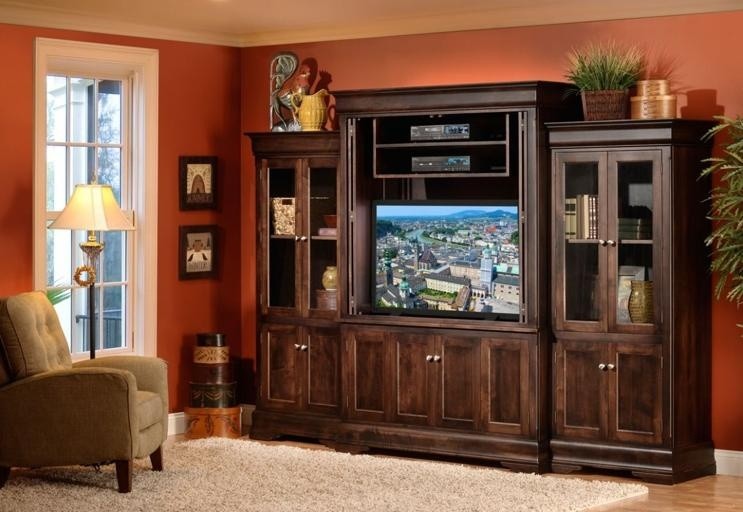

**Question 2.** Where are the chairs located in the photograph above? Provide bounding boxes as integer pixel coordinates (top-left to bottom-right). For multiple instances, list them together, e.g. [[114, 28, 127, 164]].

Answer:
[[0, 289, 172, 494]]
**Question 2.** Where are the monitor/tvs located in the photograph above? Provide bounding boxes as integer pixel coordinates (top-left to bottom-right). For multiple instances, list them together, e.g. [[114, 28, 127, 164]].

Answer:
[[370, 199, 521, 322]]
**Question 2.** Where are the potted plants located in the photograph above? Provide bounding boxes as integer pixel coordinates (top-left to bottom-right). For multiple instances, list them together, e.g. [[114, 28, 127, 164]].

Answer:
[[563, 34, 648, 121]]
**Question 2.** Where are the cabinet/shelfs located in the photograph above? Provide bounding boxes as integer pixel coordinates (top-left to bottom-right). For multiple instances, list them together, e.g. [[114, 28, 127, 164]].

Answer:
[[256, 321, 344, 419], [346, 324, 387, 425], [248, 133, 338, 319], [389, 326, 481, 432], [545, 120, 712, 334], [482, 330, 548, 439], [553, 336, 712, 452], [338, 80, 580, 332]]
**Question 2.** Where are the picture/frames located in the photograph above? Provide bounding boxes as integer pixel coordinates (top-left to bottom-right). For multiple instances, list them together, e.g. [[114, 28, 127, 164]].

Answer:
[[176, 153, 223, 283]]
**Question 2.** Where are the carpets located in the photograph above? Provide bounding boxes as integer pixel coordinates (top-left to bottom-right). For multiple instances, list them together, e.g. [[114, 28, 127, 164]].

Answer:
[[0, 433, 652, 511]]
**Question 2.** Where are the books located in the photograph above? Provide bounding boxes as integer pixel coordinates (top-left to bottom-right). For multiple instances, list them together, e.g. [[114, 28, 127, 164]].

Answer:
[[565, 193, 651, 240]]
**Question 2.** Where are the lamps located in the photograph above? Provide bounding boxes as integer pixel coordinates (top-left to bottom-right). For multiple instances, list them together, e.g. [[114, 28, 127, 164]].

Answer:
[[46, 182, 138, 361]]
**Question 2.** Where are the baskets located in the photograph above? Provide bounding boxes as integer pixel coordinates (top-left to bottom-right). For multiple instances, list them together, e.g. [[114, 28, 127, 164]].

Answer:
[[581, 90, 629, 122]]
